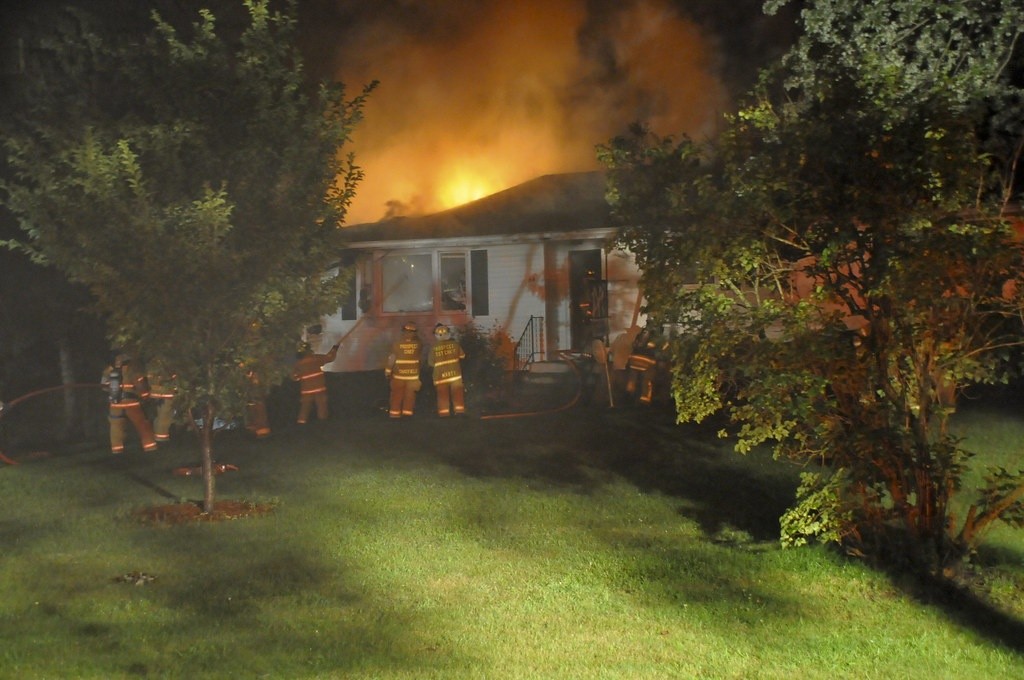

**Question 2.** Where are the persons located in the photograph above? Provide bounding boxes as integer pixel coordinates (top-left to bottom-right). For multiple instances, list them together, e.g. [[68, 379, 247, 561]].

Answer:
[[291, 342, 340, 422], [385, 321, 423, 419], [102, 349, 158, 454], [626, 328, 670, 405], [145, 350, 179, 441], [231, 348, 272, 435], [428, 325, 466, 419]]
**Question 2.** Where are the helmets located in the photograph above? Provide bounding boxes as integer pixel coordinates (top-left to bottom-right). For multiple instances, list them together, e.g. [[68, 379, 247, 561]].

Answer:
[[297, 342, 311, 352], [433, 322, 449, 336], [402, 321, 417, 332]]
